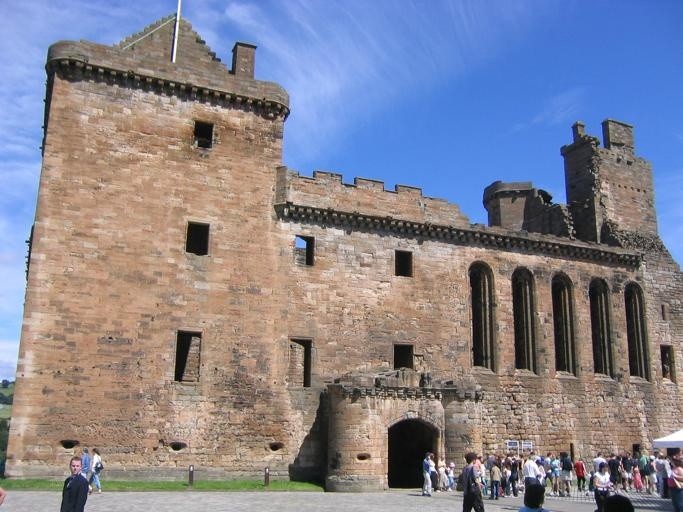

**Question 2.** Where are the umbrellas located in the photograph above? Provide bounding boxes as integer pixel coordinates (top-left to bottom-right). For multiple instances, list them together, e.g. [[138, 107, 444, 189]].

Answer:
[[652, 428, 682, 448]]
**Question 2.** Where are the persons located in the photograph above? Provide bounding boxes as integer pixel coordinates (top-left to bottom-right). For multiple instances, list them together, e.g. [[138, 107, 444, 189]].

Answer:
[[421, 447, 682, 512], [59, 456, 87, 512], [80, 447, 92, 495], [89, 447, 103, 494]]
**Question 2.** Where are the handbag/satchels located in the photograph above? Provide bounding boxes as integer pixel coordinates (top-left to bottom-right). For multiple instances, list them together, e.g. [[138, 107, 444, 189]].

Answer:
[[95, 462, 104, 473]]
